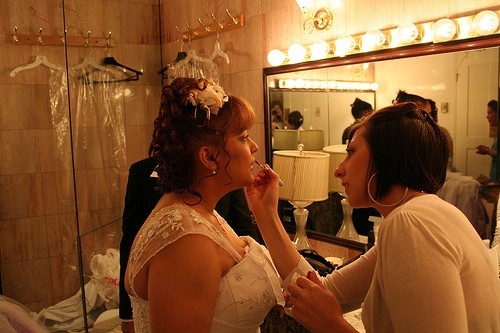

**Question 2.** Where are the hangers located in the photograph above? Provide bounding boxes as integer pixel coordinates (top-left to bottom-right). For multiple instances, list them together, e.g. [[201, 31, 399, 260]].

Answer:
[[9, 41, 65, 78], [167, 37, 215, 71], [77, 43, 143, 86], [71, 44, 122, 77], [157, 39, 202, 80], [209, 27, 230, 64]]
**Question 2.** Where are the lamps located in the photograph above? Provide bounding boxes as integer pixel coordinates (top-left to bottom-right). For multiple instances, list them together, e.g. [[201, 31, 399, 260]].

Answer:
[[299, 126, 324, 151], [322, 139, 360, 242], [272, 144, 330, 251], [273, 126, 299, 150]]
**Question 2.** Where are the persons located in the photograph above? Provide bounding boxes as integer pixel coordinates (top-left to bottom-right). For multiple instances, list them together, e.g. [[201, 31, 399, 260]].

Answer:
[[392, 91, 457, 172], [342, 98, 374, 144], [245, 102, 500, 333], [476, 100, 500, 180], [118, 77, 284, 333], [271, 100, 304, 131]]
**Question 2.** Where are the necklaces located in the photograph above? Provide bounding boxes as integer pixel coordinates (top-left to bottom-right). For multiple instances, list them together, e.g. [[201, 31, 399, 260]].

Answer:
[[399, 190, 425, 206], [182, 188, 227, 235]]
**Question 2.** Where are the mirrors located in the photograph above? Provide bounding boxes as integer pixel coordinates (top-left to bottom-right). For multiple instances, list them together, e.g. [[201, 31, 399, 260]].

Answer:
[[261, 45, 500, 265]]
